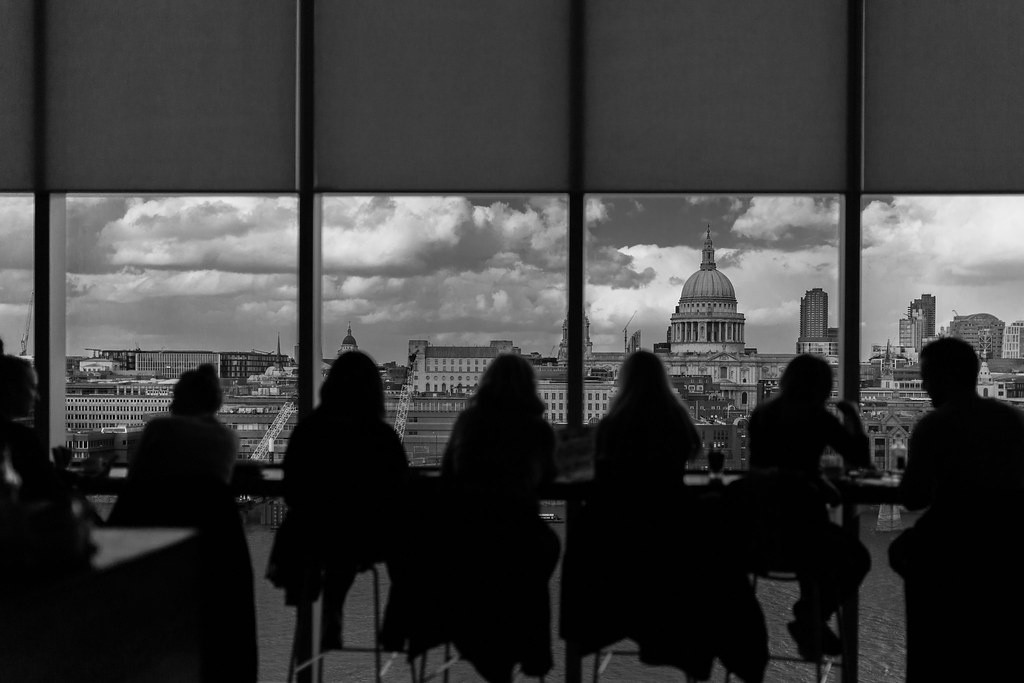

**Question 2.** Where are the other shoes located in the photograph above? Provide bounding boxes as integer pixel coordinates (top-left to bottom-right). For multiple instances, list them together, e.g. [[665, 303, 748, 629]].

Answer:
[[786, 616, 841, 664]]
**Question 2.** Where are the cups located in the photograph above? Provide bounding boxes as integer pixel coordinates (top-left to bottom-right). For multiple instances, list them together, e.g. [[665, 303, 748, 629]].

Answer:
[[83, 458, 102, 472], [707, 448, 726, 479]]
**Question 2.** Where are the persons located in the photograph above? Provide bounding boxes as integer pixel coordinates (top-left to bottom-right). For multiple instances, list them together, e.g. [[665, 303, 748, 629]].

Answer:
[[127, 362, 236, 483], [747, 354, 870, 663], [888, 339, 1024, 579], [594, 350, 702, 497], [0, 355, 74, 502], [442, 354, 561, 582], [283, 350, 409, 501]]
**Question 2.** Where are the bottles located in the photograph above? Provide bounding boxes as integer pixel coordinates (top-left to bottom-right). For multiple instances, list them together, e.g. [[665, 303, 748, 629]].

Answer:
[[888, 432, 906, 474]]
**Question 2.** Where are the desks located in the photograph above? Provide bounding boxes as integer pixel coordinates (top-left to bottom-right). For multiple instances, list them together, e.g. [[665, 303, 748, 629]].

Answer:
[[55, 460, 904, 681]]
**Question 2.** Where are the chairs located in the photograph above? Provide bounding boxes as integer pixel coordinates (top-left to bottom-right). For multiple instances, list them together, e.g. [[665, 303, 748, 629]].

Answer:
[[280, 547, 835, 682]]
